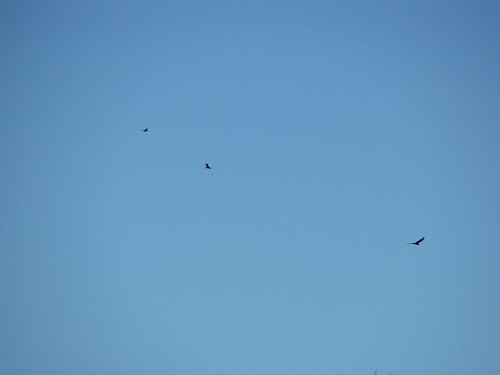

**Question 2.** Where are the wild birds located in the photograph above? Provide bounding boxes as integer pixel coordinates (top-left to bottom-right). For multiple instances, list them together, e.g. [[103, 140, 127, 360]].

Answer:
[[140, 127, 148, 133], [406, 235, 425, 245], [204, 163, 211, 170]]
